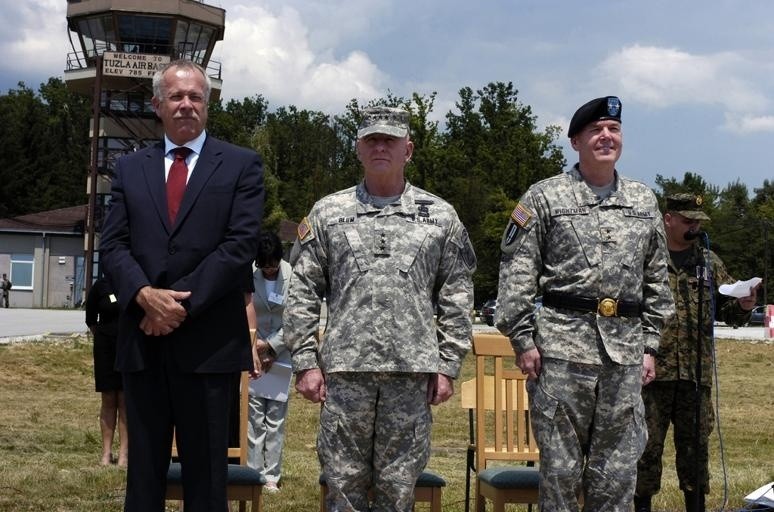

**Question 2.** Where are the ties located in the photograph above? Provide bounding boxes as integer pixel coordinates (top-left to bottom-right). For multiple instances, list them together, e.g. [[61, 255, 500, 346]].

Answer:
[[166, 149, 190, 227]]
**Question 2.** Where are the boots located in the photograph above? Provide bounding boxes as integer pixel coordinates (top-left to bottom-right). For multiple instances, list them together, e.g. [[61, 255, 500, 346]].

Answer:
[[633, 492, 652, 512], [684, 491, 706, 512]]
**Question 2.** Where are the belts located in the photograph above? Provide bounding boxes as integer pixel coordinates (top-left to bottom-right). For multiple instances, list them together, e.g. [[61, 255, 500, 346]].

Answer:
[[540, 289, 643, 317]]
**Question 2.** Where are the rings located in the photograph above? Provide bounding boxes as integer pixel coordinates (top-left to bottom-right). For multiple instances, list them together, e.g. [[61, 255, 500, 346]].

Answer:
[[521, 371, 529, 375]]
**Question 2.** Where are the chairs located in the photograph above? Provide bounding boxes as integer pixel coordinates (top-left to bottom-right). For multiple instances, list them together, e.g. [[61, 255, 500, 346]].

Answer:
[[460, 374, 538, 511], [470, 333, 587, 511], [162, 326, 269, 512], [318, 332, 446, 511]]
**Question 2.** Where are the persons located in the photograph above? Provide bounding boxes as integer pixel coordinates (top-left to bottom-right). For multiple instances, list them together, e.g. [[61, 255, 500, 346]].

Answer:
[[280, 108, 478, 512], [0, 274, 12, 308], [494, 94, 676, 512], [632, 192, 762, 512], [84, 271, 129, 469], [97, 62, 264, 512], [246, 229, 293, 495]]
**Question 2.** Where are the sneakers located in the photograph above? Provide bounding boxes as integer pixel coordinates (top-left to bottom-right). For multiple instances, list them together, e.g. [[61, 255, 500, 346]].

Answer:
[[262, 480, 279, 493]]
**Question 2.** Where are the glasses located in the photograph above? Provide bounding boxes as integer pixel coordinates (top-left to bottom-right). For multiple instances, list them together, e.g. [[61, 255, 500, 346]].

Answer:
[[254, 263, 279, 271]]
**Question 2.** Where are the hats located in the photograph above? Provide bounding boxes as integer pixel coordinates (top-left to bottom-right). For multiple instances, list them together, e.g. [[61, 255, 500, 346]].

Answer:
[[567, 94, 624, 139], [666, 191, 712, 224], [356, 105, 411, 141]]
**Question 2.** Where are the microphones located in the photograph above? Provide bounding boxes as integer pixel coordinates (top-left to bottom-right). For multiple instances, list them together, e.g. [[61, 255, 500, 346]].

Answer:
[[683, 229, 708, 241]]
